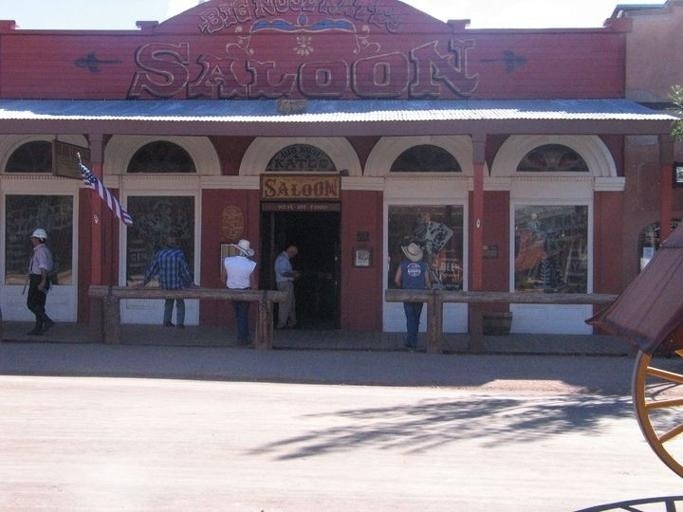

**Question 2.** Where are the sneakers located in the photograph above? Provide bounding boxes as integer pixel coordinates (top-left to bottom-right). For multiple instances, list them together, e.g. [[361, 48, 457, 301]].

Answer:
[[239, 335, 253, 344], [405, 344, 417, 351], [163, 320, 184, 328], [279, 324, 301, 329]]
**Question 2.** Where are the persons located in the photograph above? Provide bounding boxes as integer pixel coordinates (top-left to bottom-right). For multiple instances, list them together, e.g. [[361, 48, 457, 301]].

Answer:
[[413, 211, 455, 285], [394, 243, 432, 348], [223, 238, 258, 343], [128, 232, 200, 329], [25, 227, 56, 336], [273, 244, 301, 330]]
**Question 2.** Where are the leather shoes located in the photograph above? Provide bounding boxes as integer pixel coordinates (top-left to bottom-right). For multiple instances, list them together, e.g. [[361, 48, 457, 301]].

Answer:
[[32, 315, 53, 333]]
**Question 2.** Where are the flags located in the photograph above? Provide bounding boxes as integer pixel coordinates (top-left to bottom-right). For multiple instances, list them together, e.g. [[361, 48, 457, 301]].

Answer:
[[78, 162, 133, 229]]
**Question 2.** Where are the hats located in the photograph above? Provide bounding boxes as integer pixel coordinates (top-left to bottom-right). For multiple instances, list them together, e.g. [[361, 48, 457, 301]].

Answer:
[[400, 243, 423, 262], [233, 239, 255, 256], [32, 228, 48, 239]]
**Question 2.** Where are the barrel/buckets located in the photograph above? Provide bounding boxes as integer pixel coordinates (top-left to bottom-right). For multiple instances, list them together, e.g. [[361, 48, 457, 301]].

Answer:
[[484, 311, 513, 334]]
